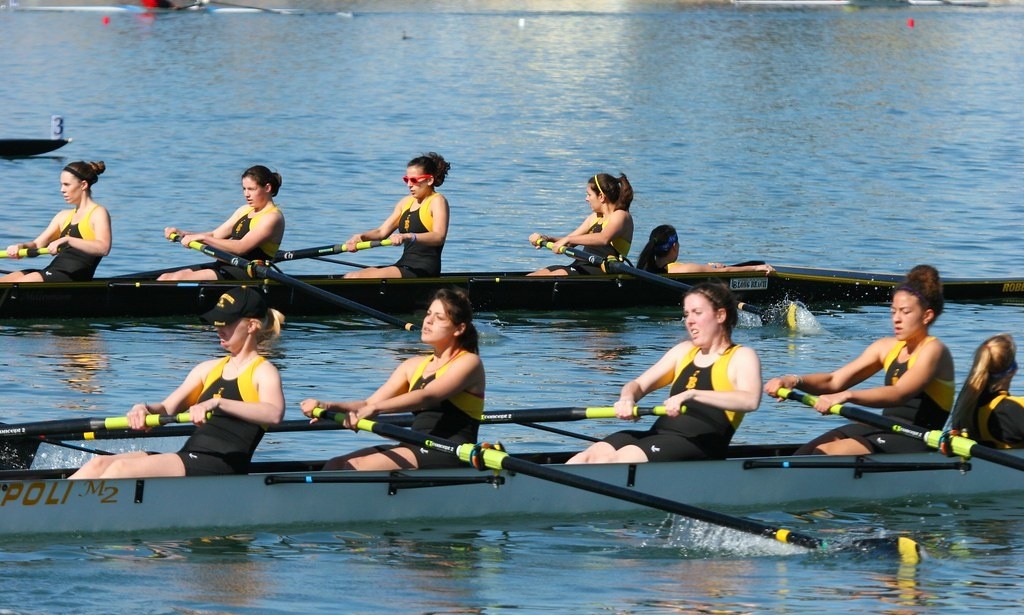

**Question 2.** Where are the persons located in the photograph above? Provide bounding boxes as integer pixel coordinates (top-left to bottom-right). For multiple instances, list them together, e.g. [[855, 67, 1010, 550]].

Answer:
[[763, 263, 956, 456], [155, 165, 285, 281], [636, 224, 776, 273], [945, 333, 1024, 450], [524, 172, 633, 276], [0, 160, 112, 284], [301, 288, 487, 472], [65, 288, 287, 480], [564, 283, 763, 465], [340, 152, 451, 278]]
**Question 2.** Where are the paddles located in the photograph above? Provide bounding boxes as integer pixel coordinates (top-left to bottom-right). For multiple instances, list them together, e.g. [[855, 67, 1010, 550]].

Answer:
[[482, 403, 687, 423], [1, 245, 62, 258], [312, 255, 386, 268], [1, 410, 214, 437], [537, 239, 805, 330], [776, 386, 1023, 472], [298, 399, 918, 576], [713, 260, 765, 266], [277, 240, 392, 262], [168, 234, 421, 333]]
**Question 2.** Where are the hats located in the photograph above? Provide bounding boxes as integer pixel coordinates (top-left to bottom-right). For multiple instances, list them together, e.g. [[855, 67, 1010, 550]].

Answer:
[[199, 286, 266, 326]]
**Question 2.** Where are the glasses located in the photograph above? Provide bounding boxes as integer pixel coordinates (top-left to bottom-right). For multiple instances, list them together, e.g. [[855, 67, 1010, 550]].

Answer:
[[401, 175, 430, 183]]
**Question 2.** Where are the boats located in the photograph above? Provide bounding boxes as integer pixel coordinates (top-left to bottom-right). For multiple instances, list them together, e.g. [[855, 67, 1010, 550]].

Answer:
[[0, 259, 1024, 319], [0, 137, 72, 159], [14, 5, 355, 20], [2, 432, 1023, 539]]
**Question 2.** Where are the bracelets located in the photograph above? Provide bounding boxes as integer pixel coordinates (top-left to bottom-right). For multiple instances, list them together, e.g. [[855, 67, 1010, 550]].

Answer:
[[785, 373, 803, 389], [408, 232, 416, 243], [708, 262, 716, 269], [543, 234, 549, 242]]
